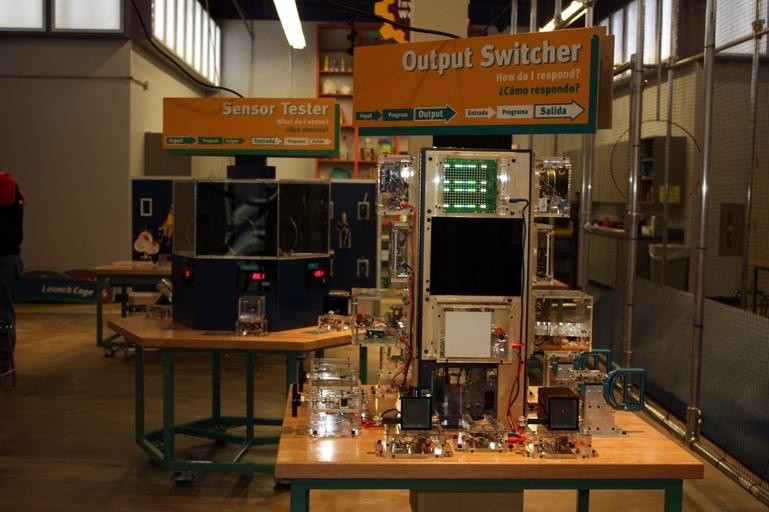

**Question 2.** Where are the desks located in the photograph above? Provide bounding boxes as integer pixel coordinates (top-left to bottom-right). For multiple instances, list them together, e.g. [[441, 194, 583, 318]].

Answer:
[[533, 287, 600, 376], [355, 312, 413, 381], [274, 377, 703, 508], [107, 308, 352, 489], [96, 264, 172, 356]]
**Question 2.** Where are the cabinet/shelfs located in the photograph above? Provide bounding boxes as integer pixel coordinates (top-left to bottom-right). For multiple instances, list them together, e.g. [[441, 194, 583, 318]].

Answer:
[[639, 136, 687, 207], [587, 234, 656, 288], [314, 23, 400, 181]]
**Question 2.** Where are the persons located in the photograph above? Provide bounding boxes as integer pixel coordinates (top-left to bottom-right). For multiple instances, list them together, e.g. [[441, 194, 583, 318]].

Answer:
[[0, 171, 23, 377]]
